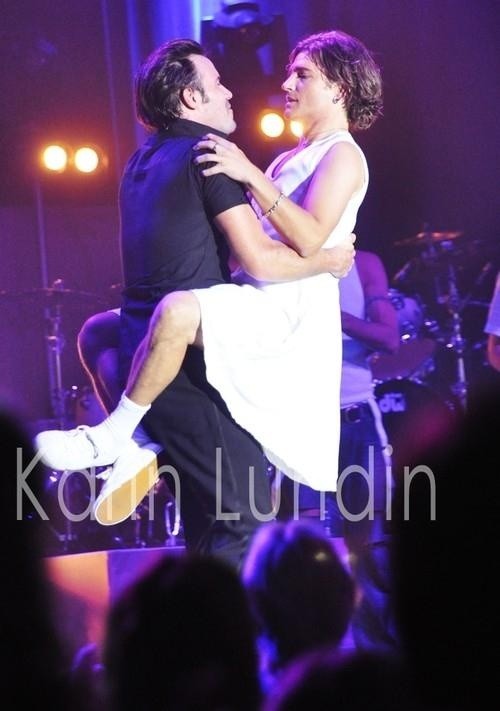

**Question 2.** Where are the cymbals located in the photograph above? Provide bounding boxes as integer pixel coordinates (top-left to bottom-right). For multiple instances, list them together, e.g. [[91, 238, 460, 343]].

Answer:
[[0, 288, 108, 310], [396, 231, 462, 247]]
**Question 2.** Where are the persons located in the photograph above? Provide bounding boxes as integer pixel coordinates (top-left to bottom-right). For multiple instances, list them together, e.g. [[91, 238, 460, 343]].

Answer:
[[32, 26, 388, 527], [475, 272, 500, 376], [0, 365, 499, 711], [116, 33, 357, 584], [266, 247, 403, 650]]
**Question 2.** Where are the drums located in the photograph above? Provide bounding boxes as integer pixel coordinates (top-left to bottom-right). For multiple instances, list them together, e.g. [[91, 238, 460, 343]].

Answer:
[[367, 287, 440, 377], [372, 371, 461, 476], [75, 385, 102, 425]]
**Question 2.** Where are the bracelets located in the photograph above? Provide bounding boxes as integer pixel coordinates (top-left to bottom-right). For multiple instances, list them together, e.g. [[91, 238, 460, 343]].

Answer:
[[260, 194, 289, 218]]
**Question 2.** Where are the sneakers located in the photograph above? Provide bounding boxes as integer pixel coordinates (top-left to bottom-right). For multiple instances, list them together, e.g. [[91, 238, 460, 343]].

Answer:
[[32, 423, 120, 471], [92, 448, 158, 525]]
